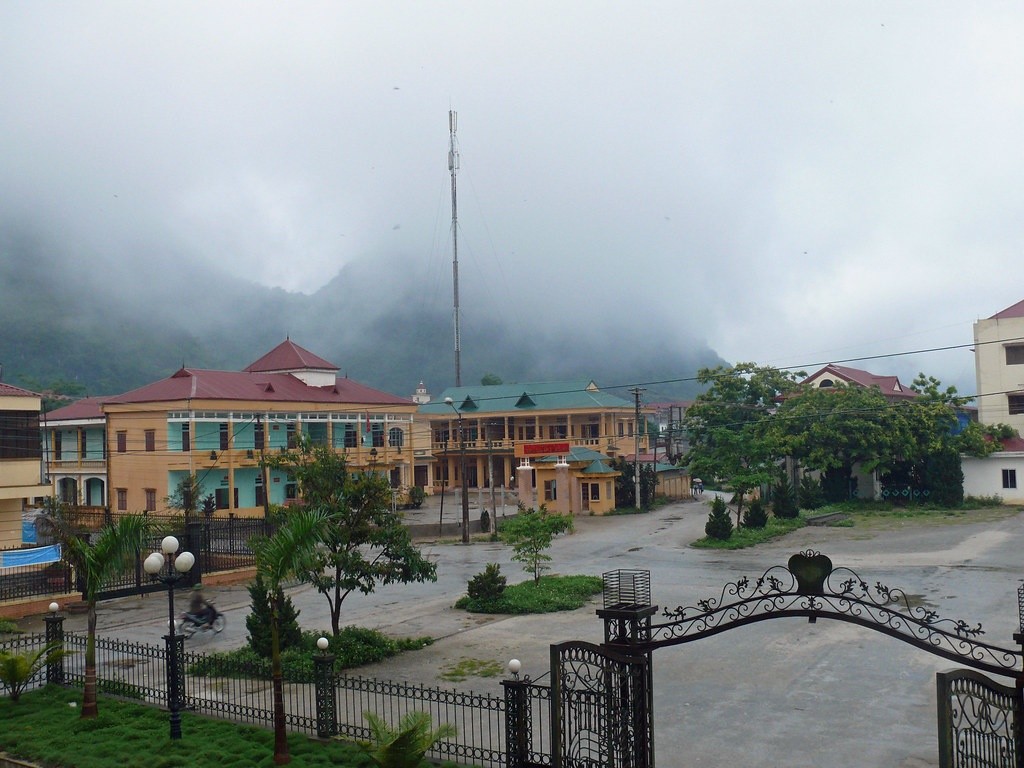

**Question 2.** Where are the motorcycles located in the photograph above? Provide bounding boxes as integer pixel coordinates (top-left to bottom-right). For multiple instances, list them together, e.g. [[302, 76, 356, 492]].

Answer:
[[176, 596, 227, 641]]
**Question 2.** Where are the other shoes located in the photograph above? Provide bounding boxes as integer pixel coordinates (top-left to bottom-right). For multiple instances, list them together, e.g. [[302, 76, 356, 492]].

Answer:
[[206, 626, 212, 629]]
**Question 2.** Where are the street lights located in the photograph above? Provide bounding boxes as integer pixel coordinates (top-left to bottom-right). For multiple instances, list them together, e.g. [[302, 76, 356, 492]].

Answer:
[[445, 397, 472, 543], [142, 535, 195, 737]]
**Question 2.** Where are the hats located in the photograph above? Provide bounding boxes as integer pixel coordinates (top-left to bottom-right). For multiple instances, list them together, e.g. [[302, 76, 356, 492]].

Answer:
[[192, 583, 203, 590]]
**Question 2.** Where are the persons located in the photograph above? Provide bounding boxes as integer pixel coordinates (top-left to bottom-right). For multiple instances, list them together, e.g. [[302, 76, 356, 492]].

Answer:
[[690, 481, 704, 496], [190, 583, 216, 629]]
